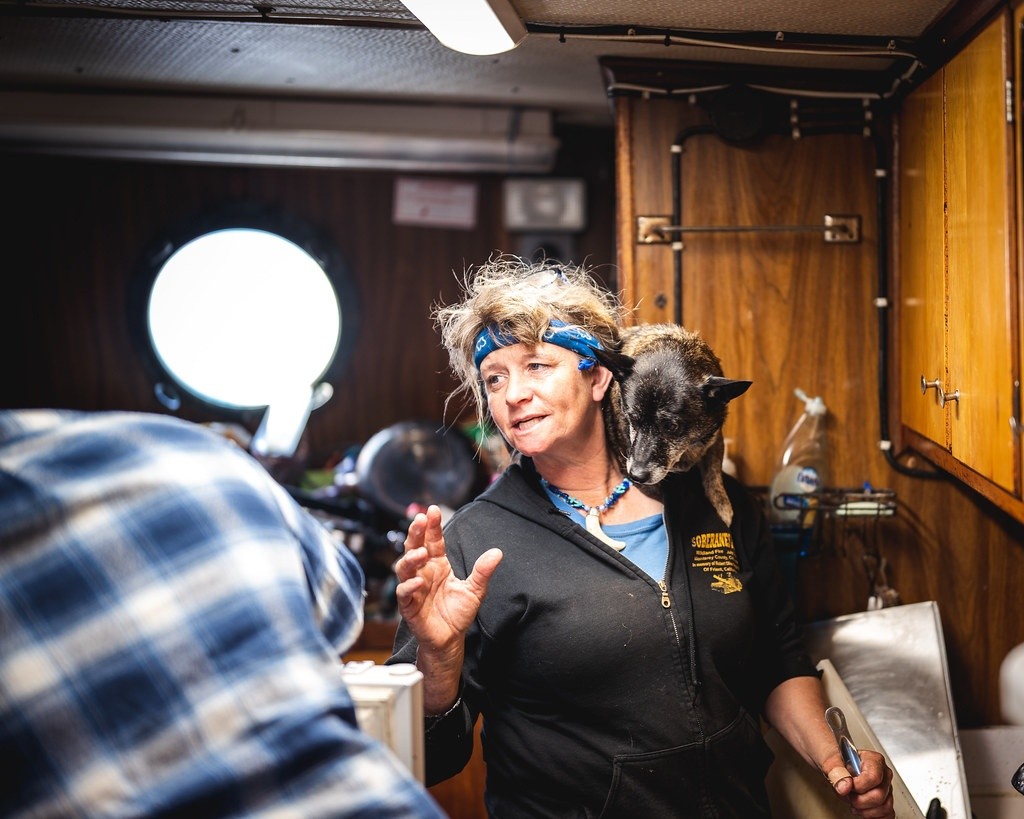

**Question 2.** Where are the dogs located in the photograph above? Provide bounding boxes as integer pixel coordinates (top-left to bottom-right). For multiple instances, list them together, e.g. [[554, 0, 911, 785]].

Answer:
[[603, 320, 754, 530]]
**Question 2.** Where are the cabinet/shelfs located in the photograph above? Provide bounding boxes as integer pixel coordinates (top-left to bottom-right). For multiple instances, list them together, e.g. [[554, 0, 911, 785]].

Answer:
[[884, 15, 1021, 515]]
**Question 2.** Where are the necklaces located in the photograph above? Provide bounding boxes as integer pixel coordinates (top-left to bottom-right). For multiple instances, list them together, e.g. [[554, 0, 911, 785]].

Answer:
[[536, 473, 633, 551]]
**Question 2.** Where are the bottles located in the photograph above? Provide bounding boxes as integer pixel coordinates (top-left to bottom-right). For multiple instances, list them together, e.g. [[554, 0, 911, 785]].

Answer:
[[721, 437, 736, 477], [763, 388, 829, 556]]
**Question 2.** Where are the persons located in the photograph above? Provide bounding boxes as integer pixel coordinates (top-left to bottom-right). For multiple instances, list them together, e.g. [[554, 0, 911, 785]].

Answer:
[[2, 403, 448, 819], [387, 253, 898, 819]]
